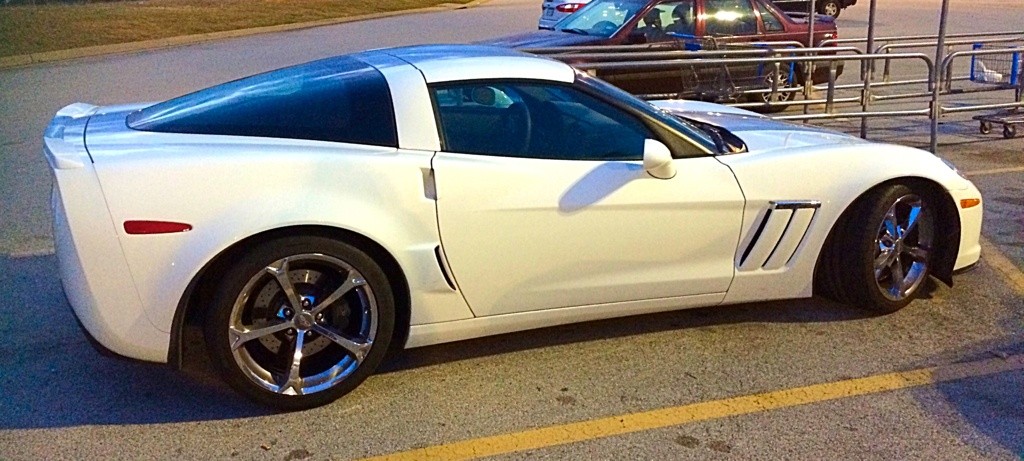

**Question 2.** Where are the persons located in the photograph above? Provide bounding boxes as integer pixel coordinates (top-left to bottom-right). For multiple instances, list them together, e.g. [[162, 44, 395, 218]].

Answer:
[[435, 94, 501, 155]]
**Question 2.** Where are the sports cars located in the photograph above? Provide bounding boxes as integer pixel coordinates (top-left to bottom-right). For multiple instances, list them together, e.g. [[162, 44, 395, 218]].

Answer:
[[40, 44, 985, 410]]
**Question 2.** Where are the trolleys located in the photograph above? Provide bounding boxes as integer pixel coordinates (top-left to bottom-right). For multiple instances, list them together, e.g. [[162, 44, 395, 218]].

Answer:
[[967, 42, 1024, 139], [664, 31, 796, 104]]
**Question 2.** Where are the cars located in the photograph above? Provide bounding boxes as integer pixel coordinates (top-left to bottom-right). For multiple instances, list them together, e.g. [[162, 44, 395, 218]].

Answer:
[[537, 0, 616, 31], [769, 0, 859, 20], [447, 1, 846, 103]]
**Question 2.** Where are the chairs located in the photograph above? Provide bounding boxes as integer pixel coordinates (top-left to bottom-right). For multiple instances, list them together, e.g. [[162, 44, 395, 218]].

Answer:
[[663, 4, 690, 37]]
